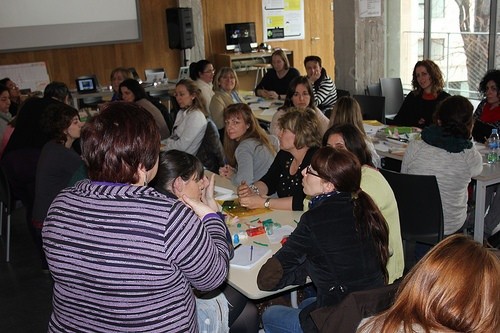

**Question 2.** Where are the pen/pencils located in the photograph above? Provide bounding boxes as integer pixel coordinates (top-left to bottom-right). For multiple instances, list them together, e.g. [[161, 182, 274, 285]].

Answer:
[[484, 137, 489, 142], [404, 131, 409, 138], [250, 246, 253, 260], [294, 220, 298, 224], [386, 136, 398, 140], [383, 142, 393, 148], [234, 244, 242, 250]]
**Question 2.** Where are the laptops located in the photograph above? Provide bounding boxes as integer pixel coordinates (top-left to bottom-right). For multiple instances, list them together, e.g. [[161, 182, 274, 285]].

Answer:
[[76, 78, 97, 93]]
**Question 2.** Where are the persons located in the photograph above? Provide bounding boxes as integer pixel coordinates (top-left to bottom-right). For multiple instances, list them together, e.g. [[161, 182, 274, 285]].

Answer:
[[470, 69, 500, 144], [401, 95, 483, 238], [0, 50, 405, 333], [356, 234, 500, 333], [389, 59, 450, 129]]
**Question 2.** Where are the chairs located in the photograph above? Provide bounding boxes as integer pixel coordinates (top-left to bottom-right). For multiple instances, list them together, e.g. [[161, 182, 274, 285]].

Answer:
[[378, 167, 444, 245], [0, 166, 24, 262], [148, 95, 181, 133], [320, 284, 396, 333], [197, 120, 226, 174], [352, 94, 386, 126], [365, 77, 404, 124]]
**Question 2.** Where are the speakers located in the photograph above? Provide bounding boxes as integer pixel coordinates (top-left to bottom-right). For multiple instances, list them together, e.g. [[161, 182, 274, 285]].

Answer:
[[166, 8, 194, 50]]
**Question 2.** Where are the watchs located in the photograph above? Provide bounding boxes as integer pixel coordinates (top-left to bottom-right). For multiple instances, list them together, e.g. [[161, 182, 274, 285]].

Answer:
[[264, 198, 272, 210]]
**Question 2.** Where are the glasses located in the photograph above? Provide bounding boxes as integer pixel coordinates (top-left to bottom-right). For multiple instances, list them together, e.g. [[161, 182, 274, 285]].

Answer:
[[306, 166, 324, 180], [173, 92, 190, 97], [204, 70, 215, 75], [10, 83, 20, 89]]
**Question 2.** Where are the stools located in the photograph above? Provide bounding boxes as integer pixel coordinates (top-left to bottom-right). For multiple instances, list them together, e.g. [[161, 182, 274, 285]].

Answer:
[[252, 63, 273, 89]]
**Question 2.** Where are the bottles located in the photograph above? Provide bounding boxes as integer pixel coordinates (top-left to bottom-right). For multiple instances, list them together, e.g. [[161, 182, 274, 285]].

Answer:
[[487, 129, 499, 164]]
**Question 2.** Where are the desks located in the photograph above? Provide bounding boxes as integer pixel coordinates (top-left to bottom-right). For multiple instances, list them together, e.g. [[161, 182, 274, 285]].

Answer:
[[203, 169, 311, 308], [250, 99, 500, 247], [69, 82, 176, 110], [216, 50, 294, 72]]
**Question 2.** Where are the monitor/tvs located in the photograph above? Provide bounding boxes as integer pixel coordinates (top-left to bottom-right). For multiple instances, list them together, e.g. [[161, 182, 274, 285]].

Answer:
[[147, 72, 163, 86], [224, 22, 258, 50]]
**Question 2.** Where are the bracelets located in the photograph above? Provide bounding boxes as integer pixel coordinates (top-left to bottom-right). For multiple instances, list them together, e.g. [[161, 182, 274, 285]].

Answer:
[[278, 94, 281, 100], [248, 185, 260, 196]]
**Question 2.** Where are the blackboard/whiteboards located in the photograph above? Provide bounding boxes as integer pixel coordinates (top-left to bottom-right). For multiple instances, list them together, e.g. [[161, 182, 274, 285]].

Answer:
[[0, 62, 51, 97]]
